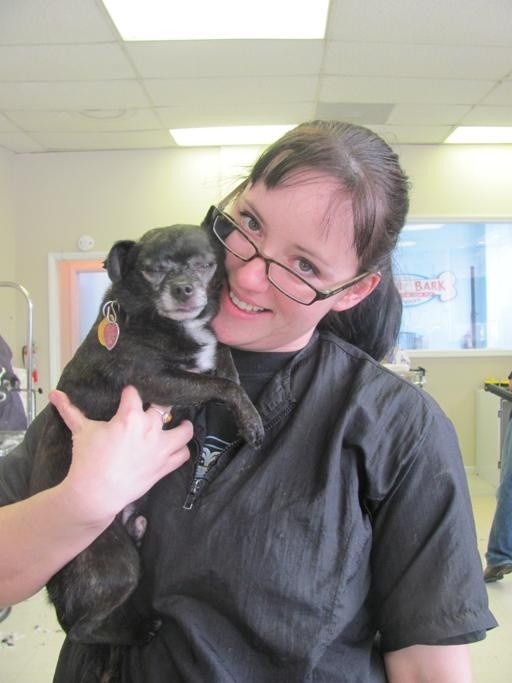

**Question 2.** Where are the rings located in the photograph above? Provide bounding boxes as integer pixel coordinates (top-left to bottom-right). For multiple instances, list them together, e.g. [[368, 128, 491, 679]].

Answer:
[[150, 405, 173, 424]]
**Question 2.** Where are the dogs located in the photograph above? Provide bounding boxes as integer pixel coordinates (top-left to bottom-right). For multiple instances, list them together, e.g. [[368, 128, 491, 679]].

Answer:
[[27, 205, 266, 683]]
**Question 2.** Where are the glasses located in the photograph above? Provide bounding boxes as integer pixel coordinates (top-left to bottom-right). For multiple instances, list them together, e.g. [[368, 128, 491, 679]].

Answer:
[[212, 177, 371, 306]]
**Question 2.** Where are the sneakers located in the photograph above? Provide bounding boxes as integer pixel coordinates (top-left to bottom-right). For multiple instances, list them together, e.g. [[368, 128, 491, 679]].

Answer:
[[484, 563, 512, 583]]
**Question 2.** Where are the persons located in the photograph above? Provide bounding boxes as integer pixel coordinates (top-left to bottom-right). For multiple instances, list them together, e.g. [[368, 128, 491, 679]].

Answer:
[[484, 369, 512, 582], [0, 119, 499, 683]]
